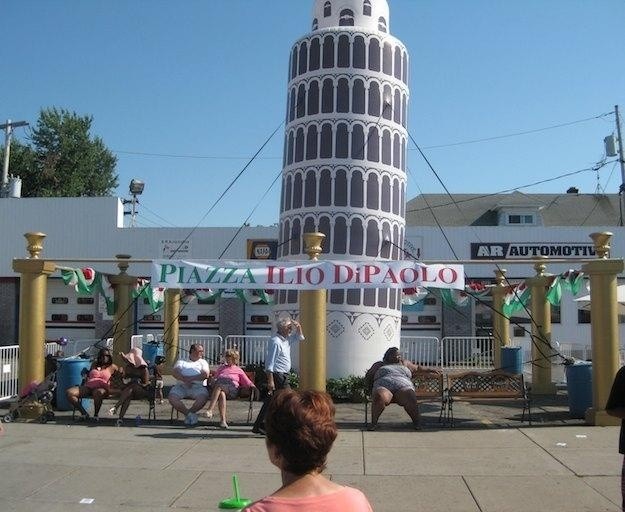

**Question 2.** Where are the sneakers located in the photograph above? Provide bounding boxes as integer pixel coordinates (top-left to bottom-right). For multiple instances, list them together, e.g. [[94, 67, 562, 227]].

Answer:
[[220, 422, 228, 428], [185, 411, 212, 424], [79, 414, 99, 423]]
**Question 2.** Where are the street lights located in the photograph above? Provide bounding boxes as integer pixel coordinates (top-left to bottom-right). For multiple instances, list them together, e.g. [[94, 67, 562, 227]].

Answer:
[[130, 178, 145, 226]]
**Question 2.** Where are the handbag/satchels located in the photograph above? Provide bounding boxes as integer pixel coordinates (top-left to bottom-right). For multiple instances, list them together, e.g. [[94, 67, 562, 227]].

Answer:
[[207, 377, 215, 397]]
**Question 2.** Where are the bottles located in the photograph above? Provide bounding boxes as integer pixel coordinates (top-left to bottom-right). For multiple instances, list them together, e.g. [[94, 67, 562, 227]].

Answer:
[[134, 414, 142, 427]]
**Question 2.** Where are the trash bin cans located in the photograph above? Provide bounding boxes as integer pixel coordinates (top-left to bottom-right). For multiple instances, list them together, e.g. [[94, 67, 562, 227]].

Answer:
[[142, 342, 165, 376], [500, 346, 523, 374], [565, 364, 593, 419], [45, 354, 91, 411]]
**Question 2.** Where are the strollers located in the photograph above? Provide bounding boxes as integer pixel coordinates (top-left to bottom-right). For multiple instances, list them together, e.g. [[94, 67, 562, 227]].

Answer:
[[1, 370, 57, 423]]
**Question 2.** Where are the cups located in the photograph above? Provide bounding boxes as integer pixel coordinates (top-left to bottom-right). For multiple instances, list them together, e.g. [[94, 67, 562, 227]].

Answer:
[[217, 498, 252, 512]]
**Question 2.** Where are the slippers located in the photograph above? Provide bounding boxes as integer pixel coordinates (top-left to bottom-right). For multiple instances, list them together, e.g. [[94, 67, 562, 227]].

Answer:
[[107, 407, 125, 427]]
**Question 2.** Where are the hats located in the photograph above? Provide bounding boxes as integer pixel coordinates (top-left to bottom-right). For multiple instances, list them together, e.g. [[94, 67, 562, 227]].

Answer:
[[119, 347, 148, 368]]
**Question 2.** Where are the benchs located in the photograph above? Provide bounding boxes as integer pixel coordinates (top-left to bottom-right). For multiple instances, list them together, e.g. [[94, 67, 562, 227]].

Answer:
[[71, 363, 255, 429], [363, 370, 532, 428]]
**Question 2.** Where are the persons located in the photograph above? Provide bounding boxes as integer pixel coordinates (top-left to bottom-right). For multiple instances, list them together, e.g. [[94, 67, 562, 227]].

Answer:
[[147, 356, 167, 404], [65, 348, 124, 423], [364, 346, 443, 432], [167, 343, 210, 427], [106, 347, 150, 427], [604, 365, 624, 512], [251, 311, 305, 435], [241, 388, 372, 512], [200, 349, 260, 431]]
